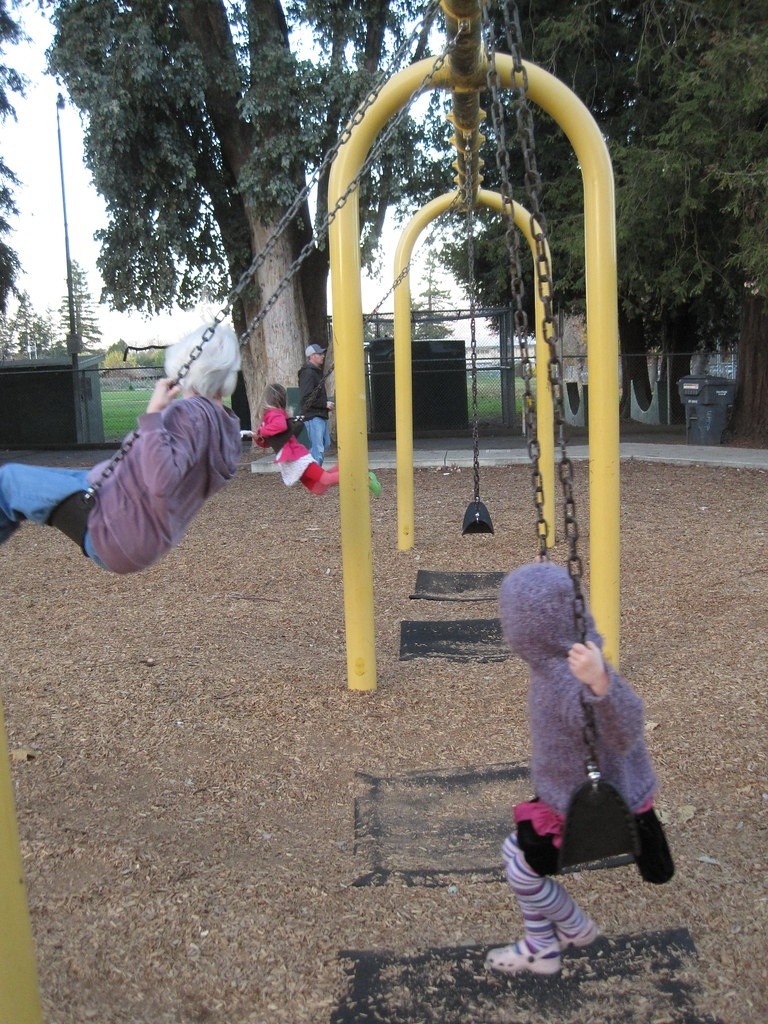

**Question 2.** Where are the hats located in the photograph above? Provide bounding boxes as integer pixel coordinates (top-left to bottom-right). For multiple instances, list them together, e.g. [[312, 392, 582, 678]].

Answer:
[[305, 343, 327, 358]]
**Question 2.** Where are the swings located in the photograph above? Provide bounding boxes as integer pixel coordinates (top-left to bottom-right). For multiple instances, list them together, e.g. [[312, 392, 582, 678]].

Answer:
[[459, 124, 496, 540], [45, 1, 472, 560], [476, 0, 642, 876], [266, 190, 473, 454]]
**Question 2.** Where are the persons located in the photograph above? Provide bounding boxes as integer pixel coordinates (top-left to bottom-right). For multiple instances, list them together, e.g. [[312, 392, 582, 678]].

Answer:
[[255, 383, 383, 499], [1, 324, 245, 573], [296, 343, 334, 468], [484, 563, 655, 974]]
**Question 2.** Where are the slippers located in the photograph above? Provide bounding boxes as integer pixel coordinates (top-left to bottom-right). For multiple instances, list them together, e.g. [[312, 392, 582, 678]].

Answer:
[[368, 472, 382, 497], [552, 919, 598, 948], [486, 937, 562, 975]]
